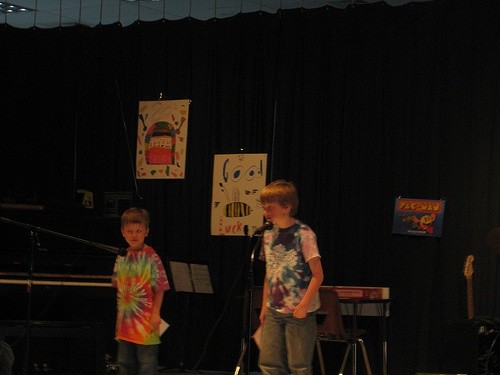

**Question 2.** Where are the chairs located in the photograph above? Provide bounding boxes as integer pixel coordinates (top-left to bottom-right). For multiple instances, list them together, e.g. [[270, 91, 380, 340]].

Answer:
[[316, 288, 373, 375]]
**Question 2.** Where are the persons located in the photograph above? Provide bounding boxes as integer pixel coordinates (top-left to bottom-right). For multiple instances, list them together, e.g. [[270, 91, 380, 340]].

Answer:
[[112, 208, 170, 375], [259, 180, 324, 375]]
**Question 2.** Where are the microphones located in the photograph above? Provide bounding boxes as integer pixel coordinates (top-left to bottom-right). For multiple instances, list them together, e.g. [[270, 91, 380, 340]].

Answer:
[[98, 244, 127, 256], [253, 222, 273, 235]]
